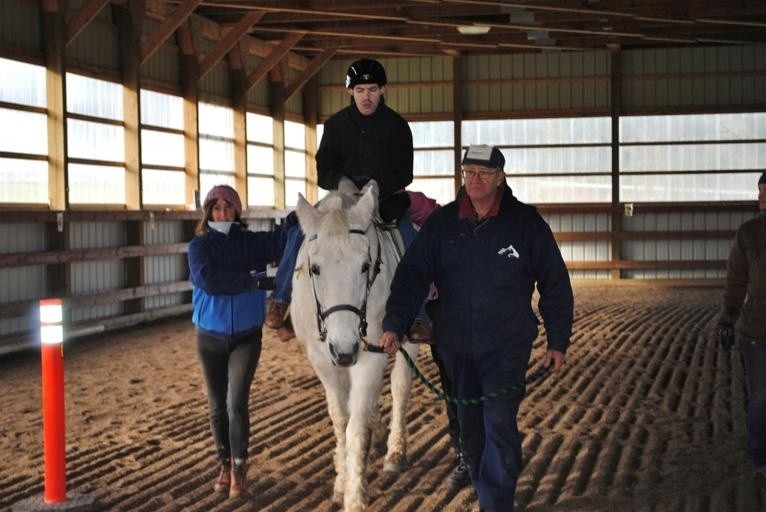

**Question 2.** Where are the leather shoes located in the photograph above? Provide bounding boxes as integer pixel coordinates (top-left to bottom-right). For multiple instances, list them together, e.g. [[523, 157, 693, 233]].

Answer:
[[447, 462, 468, 490]]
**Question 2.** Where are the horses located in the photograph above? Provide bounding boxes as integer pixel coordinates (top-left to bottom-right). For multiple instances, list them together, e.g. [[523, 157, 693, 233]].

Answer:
[[289, 186, 421, 512]]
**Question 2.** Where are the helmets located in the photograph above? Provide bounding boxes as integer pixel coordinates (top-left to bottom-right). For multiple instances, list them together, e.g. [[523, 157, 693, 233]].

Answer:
[[346, 59, 386, 89]]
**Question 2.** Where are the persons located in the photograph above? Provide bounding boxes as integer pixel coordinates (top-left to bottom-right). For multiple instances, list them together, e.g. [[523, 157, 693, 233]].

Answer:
[[376, 183, 471, 488], [262, 57, 433, 345], [184, 182, 299, 502], [714, 166, 766, 493], [375, 142, 574, 511]]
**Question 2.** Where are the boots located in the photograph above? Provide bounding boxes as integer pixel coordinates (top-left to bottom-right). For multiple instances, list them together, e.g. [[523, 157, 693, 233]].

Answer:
[[214, 459, 230, 494], [229, 457, 253, 503]]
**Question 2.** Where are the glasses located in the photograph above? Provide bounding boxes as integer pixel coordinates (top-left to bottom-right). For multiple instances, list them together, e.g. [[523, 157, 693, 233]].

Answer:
[[460, 168, 501, 180]]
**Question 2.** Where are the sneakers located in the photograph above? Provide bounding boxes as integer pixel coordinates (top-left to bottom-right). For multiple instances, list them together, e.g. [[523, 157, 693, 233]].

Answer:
[[409, 320, 431, 345], [265, 300, 290, 330]]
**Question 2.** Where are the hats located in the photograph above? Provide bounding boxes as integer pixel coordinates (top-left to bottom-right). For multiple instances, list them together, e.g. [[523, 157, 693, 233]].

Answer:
[[458, 144, 504, 173], [203, 185, 242, 217]]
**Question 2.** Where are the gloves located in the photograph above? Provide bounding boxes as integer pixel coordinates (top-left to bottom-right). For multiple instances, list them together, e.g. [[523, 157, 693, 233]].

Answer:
[[716, 319, 736, 350]]
